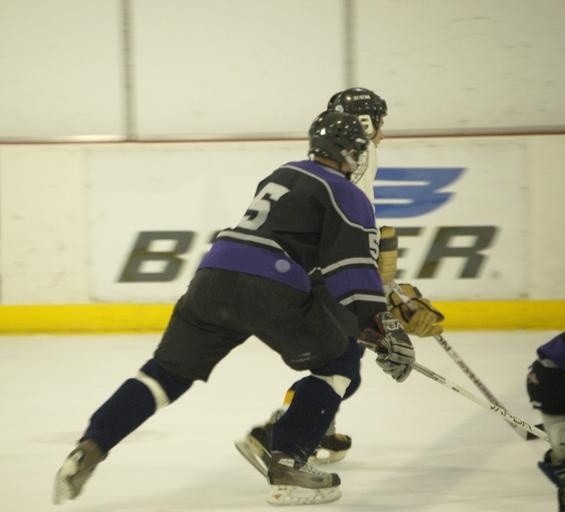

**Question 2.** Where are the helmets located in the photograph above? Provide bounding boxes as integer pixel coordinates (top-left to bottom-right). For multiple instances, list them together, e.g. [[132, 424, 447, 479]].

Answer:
[[308, 110, 370, 163], [333, 88, 388, 132]]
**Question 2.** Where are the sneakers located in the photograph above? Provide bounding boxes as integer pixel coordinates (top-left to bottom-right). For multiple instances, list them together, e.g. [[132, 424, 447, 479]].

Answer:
[[269, 449, 342, 490], [64, 440, 103, 500], [252, 408, 286, 469], [313, 432, 353, 452]]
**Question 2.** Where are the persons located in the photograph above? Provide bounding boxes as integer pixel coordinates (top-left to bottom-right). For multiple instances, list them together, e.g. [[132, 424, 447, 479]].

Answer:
[[526, 333, 565, 512], [235, 87, 445, 479], [49, 109, 415, 507]]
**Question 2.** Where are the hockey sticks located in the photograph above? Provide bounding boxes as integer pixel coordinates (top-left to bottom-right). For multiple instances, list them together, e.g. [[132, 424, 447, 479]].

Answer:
[[390, 279, 545, 440]]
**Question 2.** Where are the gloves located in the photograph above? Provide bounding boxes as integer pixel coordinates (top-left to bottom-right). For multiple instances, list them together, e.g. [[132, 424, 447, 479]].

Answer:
[[392, 282, 446, 337], [359, 312, 416, 383]]
